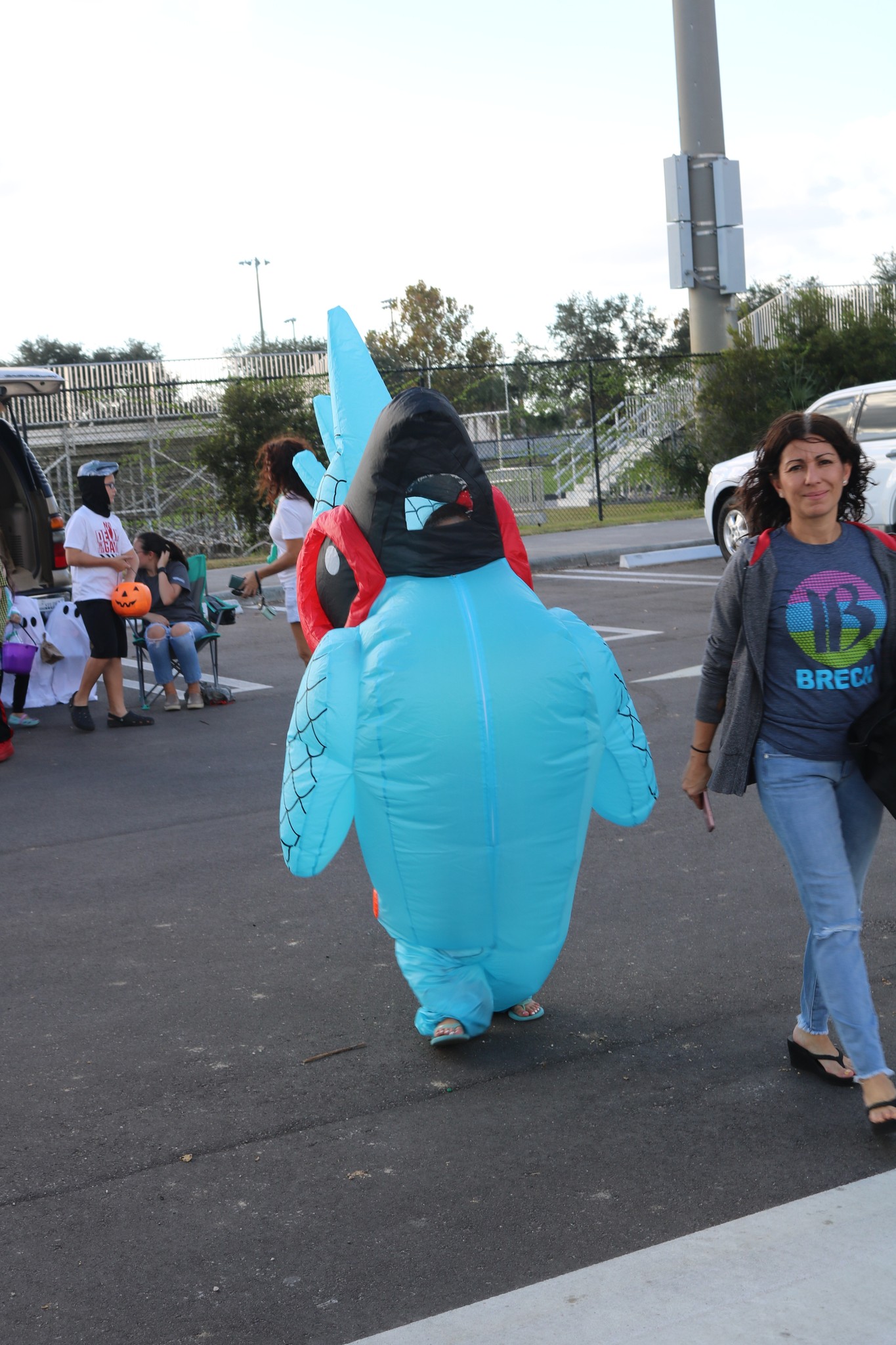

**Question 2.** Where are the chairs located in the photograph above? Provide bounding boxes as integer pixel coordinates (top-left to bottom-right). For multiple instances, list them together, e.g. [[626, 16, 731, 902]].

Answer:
[[135, 554, 220, 712]]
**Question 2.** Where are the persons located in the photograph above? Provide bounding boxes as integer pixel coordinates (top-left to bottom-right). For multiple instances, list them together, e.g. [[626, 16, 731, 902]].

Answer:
[[238, 438, 317, 667], [0, 556, 40, 761], [133, 532, 209, 710], [63, 460, 155, 731], [681, 413, 896, 1139], [279, 306, 658, 1043]]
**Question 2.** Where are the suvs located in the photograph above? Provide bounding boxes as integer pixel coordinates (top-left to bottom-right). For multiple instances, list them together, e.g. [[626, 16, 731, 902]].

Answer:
[[704, 379, 896, 563]]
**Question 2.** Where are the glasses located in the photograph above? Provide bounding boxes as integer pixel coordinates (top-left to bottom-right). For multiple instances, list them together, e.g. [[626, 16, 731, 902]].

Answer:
[[104, 481, 116, 489]]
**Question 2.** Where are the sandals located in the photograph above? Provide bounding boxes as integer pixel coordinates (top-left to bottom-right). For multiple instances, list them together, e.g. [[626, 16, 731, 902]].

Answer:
[[509, 997, 545, 1021], [431, 1017, 469, 1044], [69, 690, 95, 732], [107, 710, 155, 728]]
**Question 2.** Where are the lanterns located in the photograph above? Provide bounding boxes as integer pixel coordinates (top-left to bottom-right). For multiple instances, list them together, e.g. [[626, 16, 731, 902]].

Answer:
[[112, 582, 152, 617]]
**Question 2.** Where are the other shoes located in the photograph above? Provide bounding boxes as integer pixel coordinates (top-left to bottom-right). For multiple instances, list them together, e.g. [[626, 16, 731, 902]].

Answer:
[[7, 713, 40, 726]]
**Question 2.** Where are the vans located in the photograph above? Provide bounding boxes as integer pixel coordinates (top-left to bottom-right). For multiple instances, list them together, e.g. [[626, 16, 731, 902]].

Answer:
[[1, 366, 72, 614]]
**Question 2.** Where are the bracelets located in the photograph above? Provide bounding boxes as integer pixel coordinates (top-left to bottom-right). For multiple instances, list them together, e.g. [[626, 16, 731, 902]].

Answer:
[[156, 567, 167, 573], [690, 745, 710, 753]]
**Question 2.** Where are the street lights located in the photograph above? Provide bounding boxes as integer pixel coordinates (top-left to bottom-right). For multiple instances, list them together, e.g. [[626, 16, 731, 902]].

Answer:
[[285, 317, 297, 341], [238, 256, 271, 385], [381, 298, 397, 348]]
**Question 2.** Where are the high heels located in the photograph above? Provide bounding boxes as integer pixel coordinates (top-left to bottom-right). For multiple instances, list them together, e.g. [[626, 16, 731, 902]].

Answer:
[[785, 1035, 856, 1085], [863, 1089, 896, 1135]]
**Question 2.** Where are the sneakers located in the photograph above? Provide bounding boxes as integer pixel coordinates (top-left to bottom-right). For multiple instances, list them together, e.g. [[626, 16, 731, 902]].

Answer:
[[163, 694, 182, 710], [186, 691, 204, 708]]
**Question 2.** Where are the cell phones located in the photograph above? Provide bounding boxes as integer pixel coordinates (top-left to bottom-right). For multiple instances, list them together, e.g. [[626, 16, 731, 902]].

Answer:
[[701, 791, 715, 832]]
